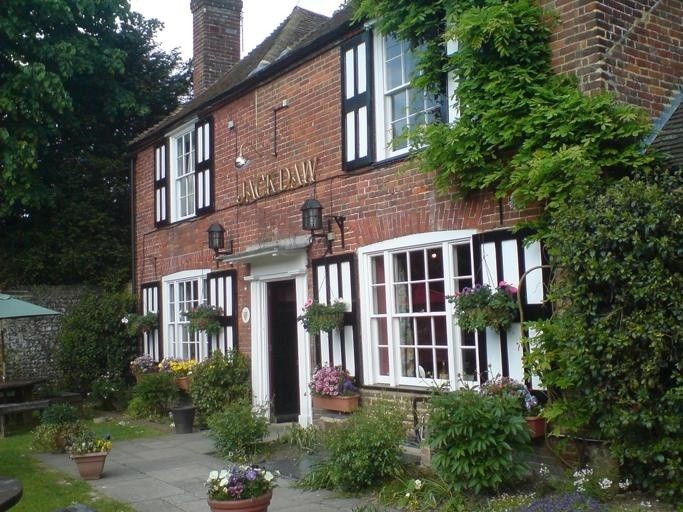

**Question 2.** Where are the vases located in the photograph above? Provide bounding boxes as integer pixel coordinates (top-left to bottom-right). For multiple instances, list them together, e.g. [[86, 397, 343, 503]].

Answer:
[[311, 394, 360, 416], [313, 313, 344, 329], [71, 452, 108, 480], [524, 417, 547, 437], [206, 490, 273, 512], [170, 406, 196, 434], [137, 376, 191, 390]]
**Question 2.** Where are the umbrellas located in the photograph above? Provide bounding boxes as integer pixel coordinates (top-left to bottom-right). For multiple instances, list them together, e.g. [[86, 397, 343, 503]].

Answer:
[[1, 295, 64, 320]]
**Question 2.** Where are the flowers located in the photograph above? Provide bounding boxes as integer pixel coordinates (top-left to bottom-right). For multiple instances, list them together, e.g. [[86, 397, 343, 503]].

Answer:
[[120, 312, 158, 337], [180, 304, 223, 336], [296, 298, 345, 337], [309, 363, 354, 396], [129, 353, 196, 376], [203, 465, 274, 501], [447, 281, 517, 334], [478, 378, 538, 411], [65, 431, 112, 456]]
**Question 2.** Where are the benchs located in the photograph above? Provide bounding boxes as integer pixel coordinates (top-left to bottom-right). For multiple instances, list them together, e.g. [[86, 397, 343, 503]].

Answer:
[[51, 393, 85, 416], [0, 399, 52, 437]]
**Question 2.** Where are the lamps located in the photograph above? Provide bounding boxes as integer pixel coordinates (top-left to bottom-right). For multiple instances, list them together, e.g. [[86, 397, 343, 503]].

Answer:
[[235, 99, 289, 169], [299, 198, 344, 248], [207, 224, 234, 257]]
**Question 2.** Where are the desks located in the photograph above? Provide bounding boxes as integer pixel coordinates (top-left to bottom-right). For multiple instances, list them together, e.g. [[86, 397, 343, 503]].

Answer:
[[0, 380, 46, 402]]
